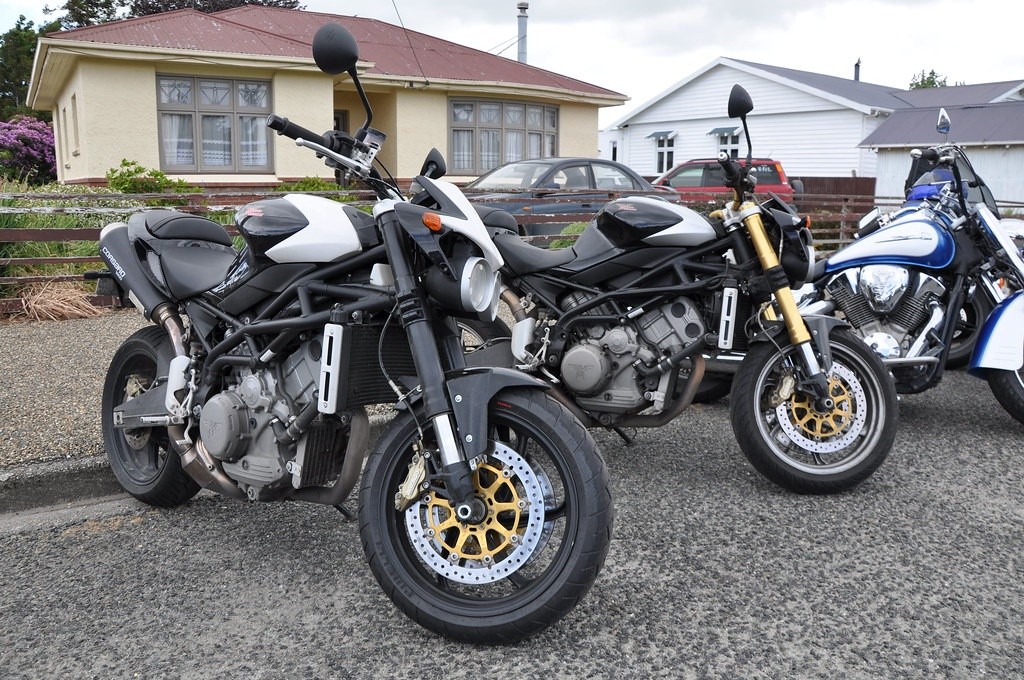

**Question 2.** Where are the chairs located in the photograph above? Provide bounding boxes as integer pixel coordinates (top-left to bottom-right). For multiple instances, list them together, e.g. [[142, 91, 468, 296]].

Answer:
[[538, 174, 633, 189]]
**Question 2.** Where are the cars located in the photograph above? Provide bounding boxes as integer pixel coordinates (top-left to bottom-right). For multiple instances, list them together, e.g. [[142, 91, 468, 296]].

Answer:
[[456, 156, 659, 224]]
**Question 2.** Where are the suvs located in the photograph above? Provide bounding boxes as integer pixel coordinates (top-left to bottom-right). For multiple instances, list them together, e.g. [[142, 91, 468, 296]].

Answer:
[[648, 155, 804, 219]]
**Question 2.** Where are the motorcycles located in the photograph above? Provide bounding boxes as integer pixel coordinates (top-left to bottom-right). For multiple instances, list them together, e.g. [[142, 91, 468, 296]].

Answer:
[[430, 82, 902, 494], [85, 22, 618, 646], [689, 107, 1023, 423]]
